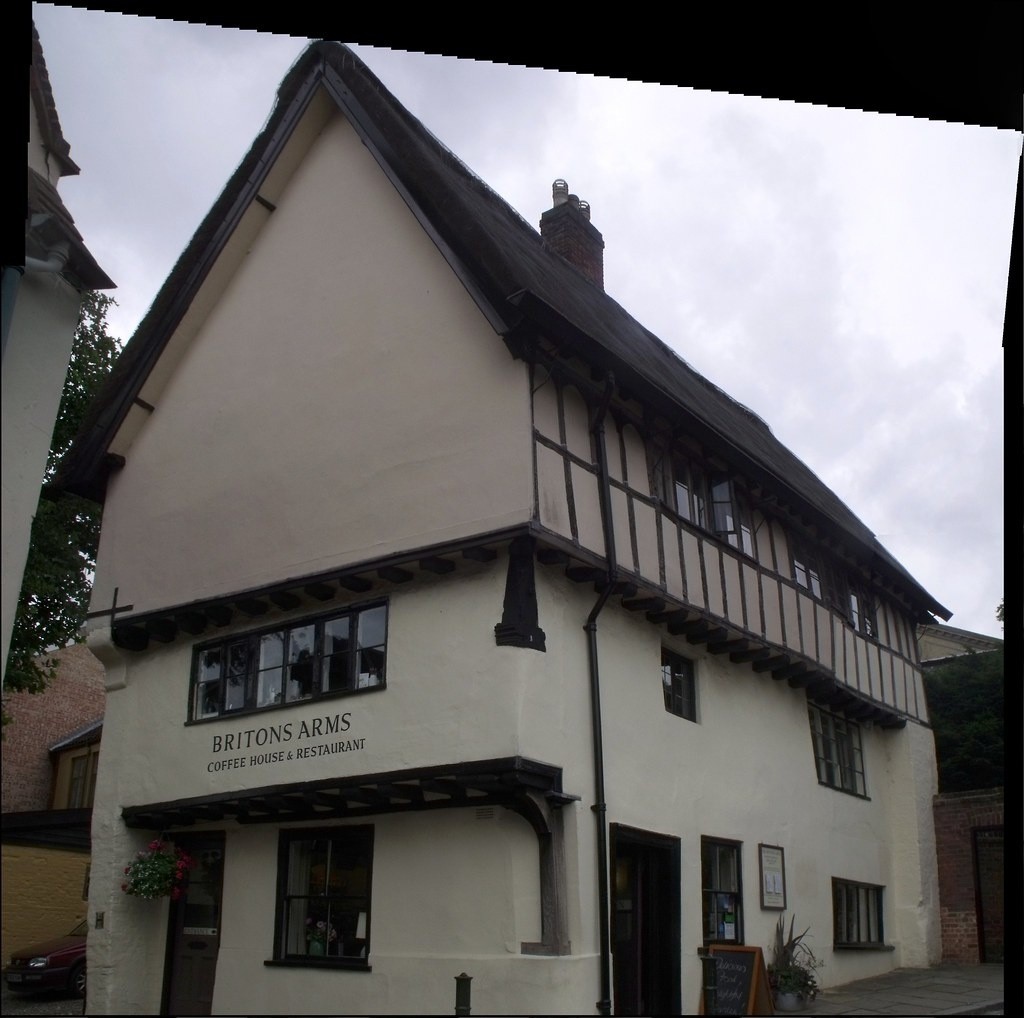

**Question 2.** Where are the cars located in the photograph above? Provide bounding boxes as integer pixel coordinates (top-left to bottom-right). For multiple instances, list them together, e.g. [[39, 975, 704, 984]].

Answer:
[[3, 921, 85, 998]]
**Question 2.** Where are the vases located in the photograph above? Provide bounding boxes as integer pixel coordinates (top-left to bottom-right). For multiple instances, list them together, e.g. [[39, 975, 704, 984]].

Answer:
[[308, 940, 324, 957]]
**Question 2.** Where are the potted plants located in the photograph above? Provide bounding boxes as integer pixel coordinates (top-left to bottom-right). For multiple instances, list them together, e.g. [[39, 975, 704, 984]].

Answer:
[[767, 962, 821, 1011]]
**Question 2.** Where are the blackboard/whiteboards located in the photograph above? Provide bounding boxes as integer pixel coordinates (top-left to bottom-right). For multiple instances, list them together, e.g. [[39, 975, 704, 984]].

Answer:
[[697, 945, 775, 1016]]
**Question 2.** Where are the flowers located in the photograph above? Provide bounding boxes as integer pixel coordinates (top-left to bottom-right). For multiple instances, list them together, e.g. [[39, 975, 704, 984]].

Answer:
[[121, 839, 193, 900], [305, 917, 336, 943]]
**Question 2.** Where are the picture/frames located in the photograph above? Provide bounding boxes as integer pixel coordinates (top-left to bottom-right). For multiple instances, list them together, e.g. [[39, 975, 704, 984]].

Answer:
[[757, 843, 787, 910]]
